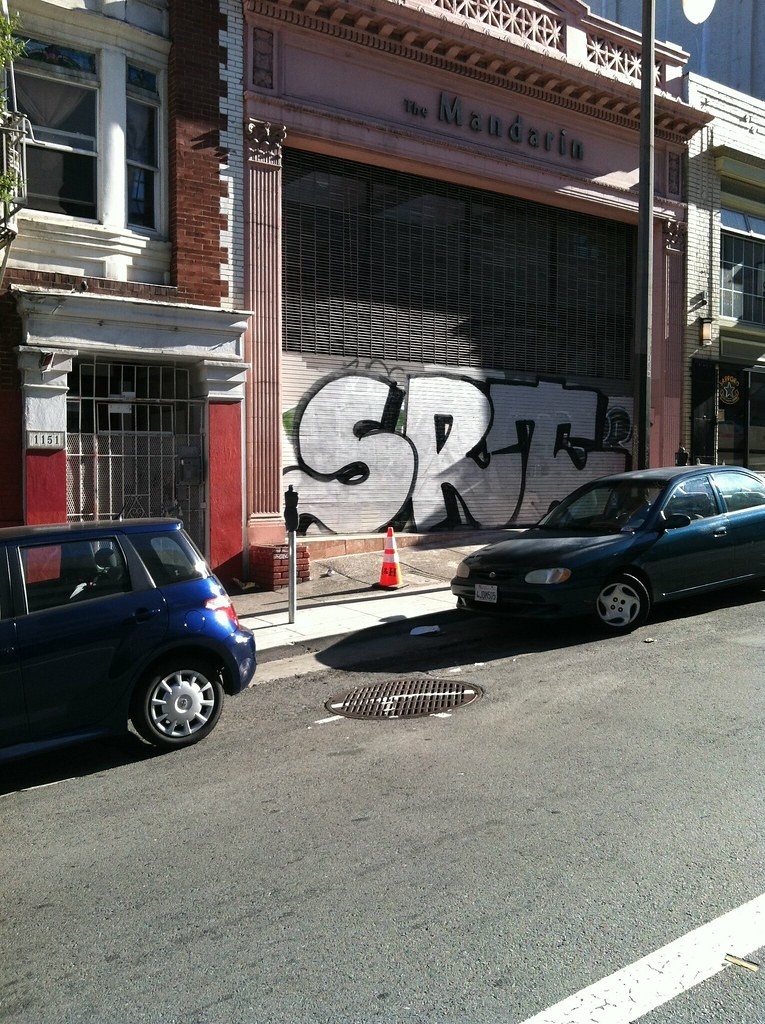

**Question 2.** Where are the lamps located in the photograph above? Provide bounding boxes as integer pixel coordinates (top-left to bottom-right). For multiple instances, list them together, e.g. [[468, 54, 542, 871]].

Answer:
[[700, 318, 716, 347]]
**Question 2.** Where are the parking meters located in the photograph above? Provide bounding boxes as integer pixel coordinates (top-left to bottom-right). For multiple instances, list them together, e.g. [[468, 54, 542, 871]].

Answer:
[[674, 447, 689, 466], [284, 484, 300, 624]]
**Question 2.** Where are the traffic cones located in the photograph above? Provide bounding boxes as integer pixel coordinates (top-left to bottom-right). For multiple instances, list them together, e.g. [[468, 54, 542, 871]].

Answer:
[[371, 526, 409, 591]]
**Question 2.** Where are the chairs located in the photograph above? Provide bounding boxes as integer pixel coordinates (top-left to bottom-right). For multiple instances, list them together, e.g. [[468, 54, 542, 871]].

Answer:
[[91, 548, 125, 590]]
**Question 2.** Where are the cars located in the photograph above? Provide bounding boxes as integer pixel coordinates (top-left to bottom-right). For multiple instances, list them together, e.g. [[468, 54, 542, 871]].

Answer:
[[0, 517, 257, 765], [452, 464, 765, 633]]
